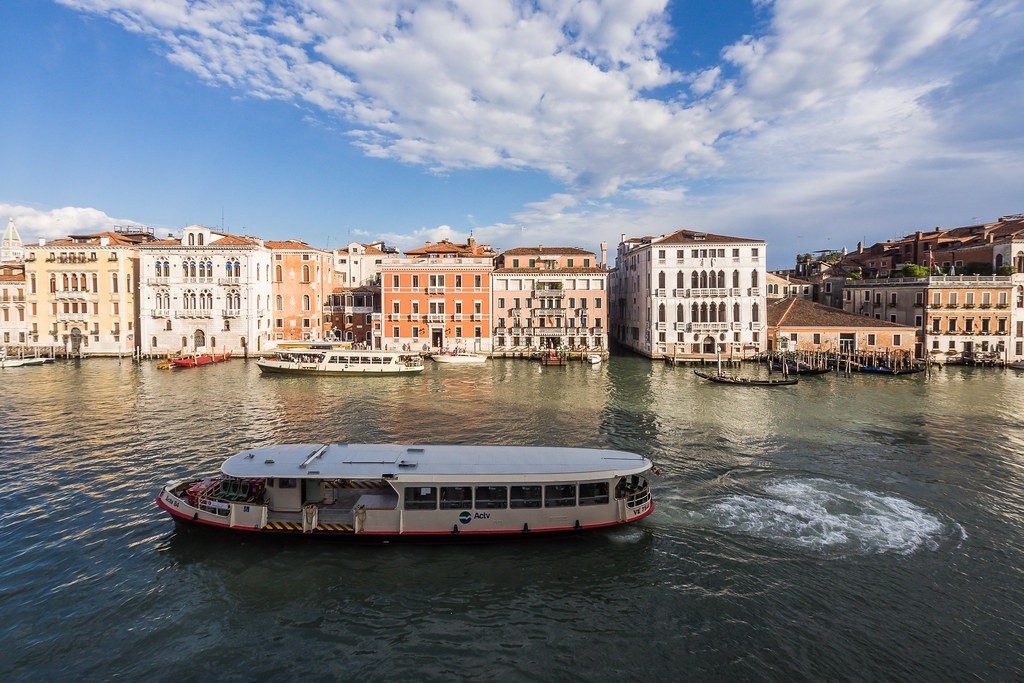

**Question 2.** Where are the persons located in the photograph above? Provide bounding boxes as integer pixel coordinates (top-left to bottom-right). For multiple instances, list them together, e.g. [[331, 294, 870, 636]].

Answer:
[[270, 353, 325, 363], [401, 355, 423, 364], [402, 343, 407, 351], [385, 344, 387, 350]]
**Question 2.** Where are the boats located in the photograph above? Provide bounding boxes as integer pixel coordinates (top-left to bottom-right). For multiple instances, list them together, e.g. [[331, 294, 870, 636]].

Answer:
[[253, 340, 425, 375], [693, 369, 799, 386], [430, 352, 487, 364], [772, 361, 835, 375], [831, 362, 925, 375], [156, 441, 663, 545], [586, 354, 602, 365], [172, 352, 232, 368]]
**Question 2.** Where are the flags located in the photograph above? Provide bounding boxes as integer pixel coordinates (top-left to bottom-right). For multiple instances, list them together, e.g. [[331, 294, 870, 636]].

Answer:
[[651, 465, 661, 476], [930, 250, 935, 268]]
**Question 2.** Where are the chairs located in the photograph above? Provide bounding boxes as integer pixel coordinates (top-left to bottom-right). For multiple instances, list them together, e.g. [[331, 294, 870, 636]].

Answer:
[[617, 475, 648, 508], [217, 477, 266, 507]]
